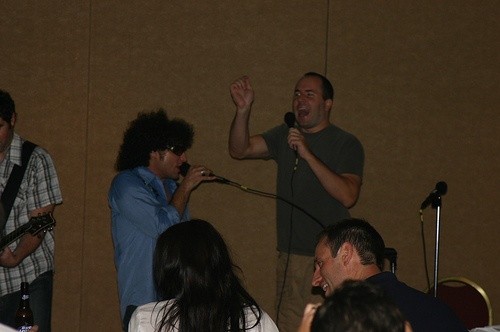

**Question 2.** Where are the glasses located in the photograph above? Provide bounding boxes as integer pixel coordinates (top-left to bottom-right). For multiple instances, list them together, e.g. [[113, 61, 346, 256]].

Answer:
[[166, 144, 186, 156]]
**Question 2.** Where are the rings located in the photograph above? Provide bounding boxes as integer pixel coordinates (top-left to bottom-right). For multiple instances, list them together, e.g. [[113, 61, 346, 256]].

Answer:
[[200, 169, 206, 176]]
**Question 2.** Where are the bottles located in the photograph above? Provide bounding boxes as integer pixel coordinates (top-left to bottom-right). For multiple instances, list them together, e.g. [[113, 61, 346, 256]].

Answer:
[[16, 282, 34, 332]]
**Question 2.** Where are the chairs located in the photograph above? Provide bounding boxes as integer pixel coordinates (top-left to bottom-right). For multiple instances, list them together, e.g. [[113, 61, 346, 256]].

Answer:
[[419, 278, 492, 332]]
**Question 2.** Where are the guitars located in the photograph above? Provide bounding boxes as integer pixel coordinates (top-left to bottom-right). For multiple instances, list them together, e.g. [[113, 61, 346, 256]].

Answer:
[[0, 211, 56, 251]]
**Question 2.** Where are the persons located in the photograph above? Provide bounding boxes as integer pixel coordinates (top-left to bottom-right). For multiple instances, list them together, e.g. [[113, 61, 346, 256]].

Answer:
[[310, 287, 413, 331], [126, 218, 279, 332], [0, 89, 63, 332], [0, 322, 39, 332], [108, 110, 216, 332], [227, 72, 365, 332], [297, 218, 467, 332]]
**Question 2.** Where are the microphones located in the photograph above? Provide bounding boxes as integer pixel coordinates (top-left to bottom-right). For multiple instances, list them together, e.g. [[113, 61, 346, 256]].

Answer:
[[420, 181, 447, 210], [179, 162, 227, 185], [284, 112, 298, 156]]
